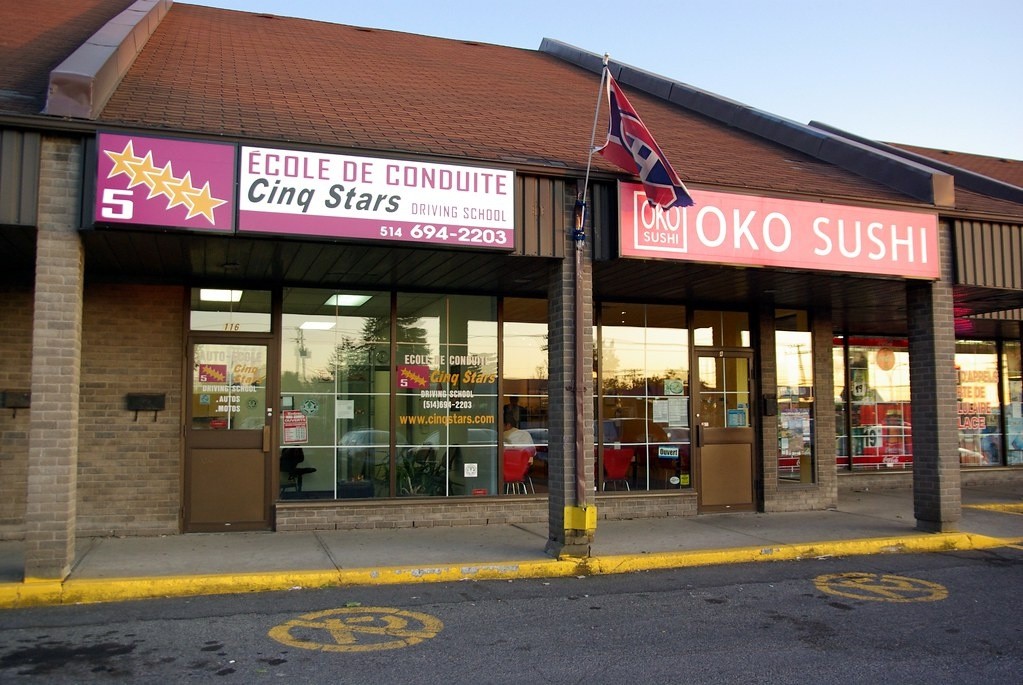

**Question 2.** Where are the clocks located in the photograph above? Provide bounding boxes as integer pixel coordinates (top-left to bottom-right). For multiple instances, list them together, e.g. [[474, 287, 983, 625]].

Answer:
[[376, 351, 390, 364]]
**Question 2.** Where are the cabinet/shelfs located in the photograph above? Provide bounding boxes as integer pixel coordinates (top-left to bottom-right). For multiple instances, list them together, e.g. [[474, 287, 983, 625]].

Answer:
[[192, 393, 236, 429]]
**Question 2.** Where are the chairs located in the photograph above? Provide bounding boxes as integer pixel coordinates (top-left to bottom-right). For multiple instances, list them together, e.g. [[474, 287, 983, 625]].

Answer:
[[441, 444, 459, 495], [630, 445, 656, 490], [502, 447, 531, 494], [679, 445, 689, 489], [282, 445, 319, 499], [594, 448, 598, 486], [521, 447, 536, 494], [415, 442, 432, 464], [657, 444, 676, 489], [602, 448, 635, 491]]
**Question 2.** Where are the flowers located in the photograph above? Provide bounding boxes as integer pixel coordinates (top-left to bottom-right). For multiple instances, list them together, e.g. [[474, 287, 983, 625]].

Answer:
[[352, 473, 363, 482]]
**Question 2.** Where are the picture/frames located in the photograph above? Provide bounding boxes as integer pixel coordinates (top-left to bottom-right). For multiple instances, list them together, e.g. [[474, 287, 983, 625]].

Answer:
[[604, 389, 617, 406]]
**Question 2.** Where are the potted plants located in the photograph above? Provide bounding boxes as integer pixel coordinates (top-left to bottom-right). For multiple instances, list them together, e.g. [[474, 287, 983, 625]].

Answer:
[[373, 447, 464, 497]]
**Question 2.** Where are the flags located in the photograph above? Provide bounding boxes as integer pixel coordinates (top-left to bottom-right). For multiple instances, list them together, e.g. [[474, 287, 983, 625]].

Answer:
[[589, 65, 695, 211]]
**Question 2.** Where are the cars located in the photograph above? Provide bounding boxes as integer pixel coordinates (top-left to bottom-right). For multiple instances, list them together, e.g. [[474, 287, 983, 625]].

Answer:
[[522, 427, 548, 474], [835, 426, 983, 467], [592, 416, 690, 473], [339, 427, 410, 468], [406, 428, 501, 487]]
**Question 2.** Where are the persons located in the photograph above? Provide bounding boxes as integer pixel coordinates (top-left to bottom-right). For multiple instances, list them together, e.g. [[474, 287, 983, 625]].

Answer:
[[502, 418, 537, 469], [594, 415, 618, 443], [503, 394, 528, 430]]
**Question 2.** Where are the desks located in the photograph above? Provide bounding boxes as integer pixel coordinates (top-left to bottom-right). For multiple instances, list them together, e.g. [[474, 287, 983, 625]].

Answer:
[[595, 456, 636, 491]]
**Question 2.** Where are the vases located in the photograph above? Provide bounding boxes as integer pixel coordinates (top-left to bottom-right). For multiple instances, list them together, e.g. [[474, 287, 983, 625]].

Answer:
[[336, 482, 373, 498]]
